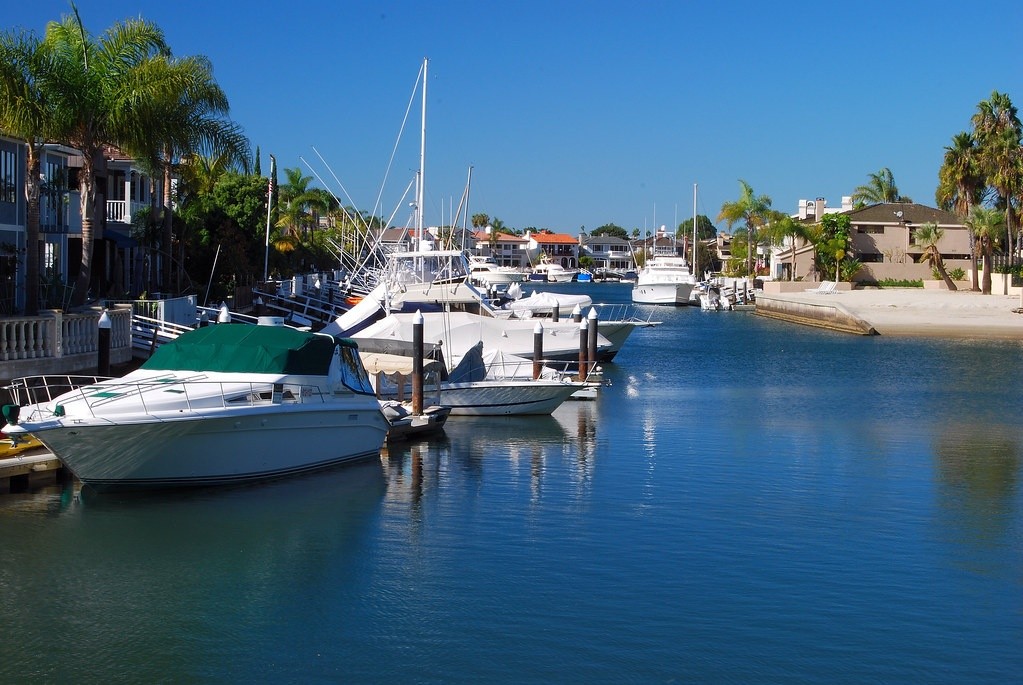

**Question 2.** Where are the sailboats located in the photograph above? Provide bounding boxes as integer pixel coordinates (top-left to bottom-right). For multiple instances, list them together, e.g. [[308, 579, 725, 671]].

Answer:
[[248, 55, 665, 417], [630, 181, 757, 312]]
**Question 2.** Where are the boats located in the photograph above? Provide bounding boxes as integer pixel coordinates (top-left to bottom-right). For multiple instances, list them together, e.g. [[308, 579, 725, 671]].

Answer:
[[470, 251, 637, 289], [1, 321, 389, 485]]
